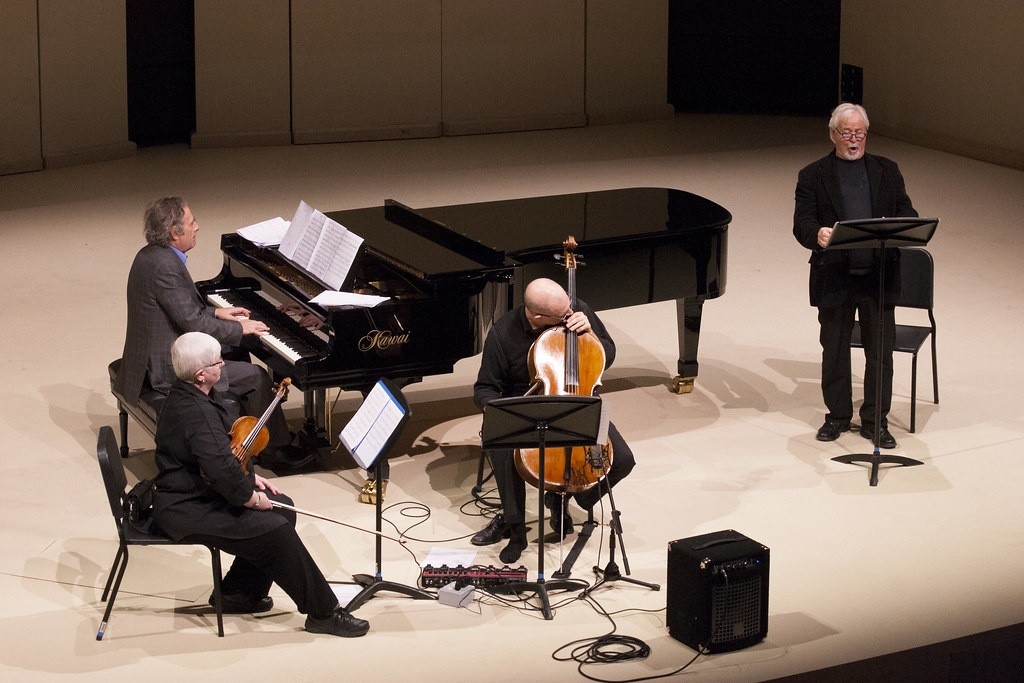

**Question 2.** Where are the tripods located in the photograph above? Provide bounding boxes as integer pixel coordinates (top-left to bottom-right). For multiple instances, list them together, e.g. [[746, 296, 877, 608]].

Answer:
[[339, 377, 439, 612], [823, 218, 938, 486], [597, 464, 662, 600], [488, 395, 602, 621]]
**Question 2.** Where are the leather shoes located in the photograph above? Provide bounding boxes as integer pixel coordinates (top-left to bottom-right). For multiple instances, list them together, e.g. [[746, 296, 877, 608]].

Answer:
[[817, 421, 849, 441], [289, 431, 296, 444], [209, 588, 273, 613], [305, 607, 369, 636], [259, 445, 320, 469], [860, 422, 896, 448], [546, 492, 573, 534], [471, 513, 510, 545]]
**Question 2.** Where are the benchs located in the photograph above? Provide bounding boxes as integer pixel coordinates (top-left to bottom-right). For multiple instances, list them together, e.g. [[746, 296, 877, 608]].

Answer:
[[109, 358, 169, 458]]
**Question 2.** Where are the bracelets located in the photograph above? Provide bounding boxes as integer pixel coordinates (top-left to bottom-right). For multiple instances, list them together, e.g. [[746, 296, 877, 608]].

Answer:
[[251, 492, 261, 510]]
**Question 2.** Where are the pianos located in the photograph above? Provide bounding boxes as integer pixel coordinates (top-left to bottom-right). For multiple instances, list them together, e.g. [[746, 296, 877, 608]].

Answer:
[[195, 187, 744, 506]]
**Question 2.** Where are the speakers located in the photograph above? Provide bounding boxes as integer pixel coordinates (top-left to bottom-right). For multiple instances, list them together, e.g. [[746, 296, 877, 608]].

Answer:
[[665, 528, 771, 655]]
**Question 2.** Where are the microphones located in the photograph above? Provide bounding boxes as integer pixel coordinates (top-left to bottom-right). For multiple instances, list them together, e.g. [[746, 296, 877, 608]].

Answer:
[[589, 444, 602, 469]]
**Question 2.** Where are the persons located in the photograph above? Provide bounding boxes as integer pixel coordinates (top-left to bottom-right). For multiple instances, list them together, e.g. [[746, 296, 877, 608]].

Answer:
[[113, 196, 317, 472], [150, 332, 370, 637], [793, 103, 920, 449], [470, 278, 636, 564]]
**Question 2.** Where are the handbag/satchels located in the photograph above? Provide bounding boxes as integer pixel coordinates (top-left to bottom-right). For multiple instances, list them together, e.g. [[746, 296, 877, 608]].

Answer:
[[124, 479, 155, 534]]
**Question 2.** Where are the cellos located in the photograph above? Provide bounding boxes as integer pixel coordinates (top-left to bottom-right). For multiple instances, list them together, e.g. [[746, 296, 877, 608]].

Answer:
[[513, 235, 614, 576]]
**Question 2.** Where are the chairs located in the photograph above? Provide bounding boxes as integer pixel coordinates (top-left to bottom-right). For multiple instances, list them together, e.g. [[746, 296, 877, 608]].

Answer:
[[851, 247, 939, 432], [96, 425, 225, 640]]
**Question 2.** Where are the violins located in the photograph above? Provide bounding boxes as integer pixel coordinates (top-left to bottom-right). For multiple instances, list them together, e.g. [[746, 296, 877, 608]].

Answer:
[[228, 377, 292, 474]]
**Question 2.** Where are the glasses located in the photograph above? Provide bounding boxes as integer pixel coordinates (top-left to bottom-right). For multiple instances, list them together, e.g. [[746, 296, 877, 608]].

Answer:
[[836, 128, 868, 141], [194, 357, 224, 373], [536, 292, 573, 321]]
